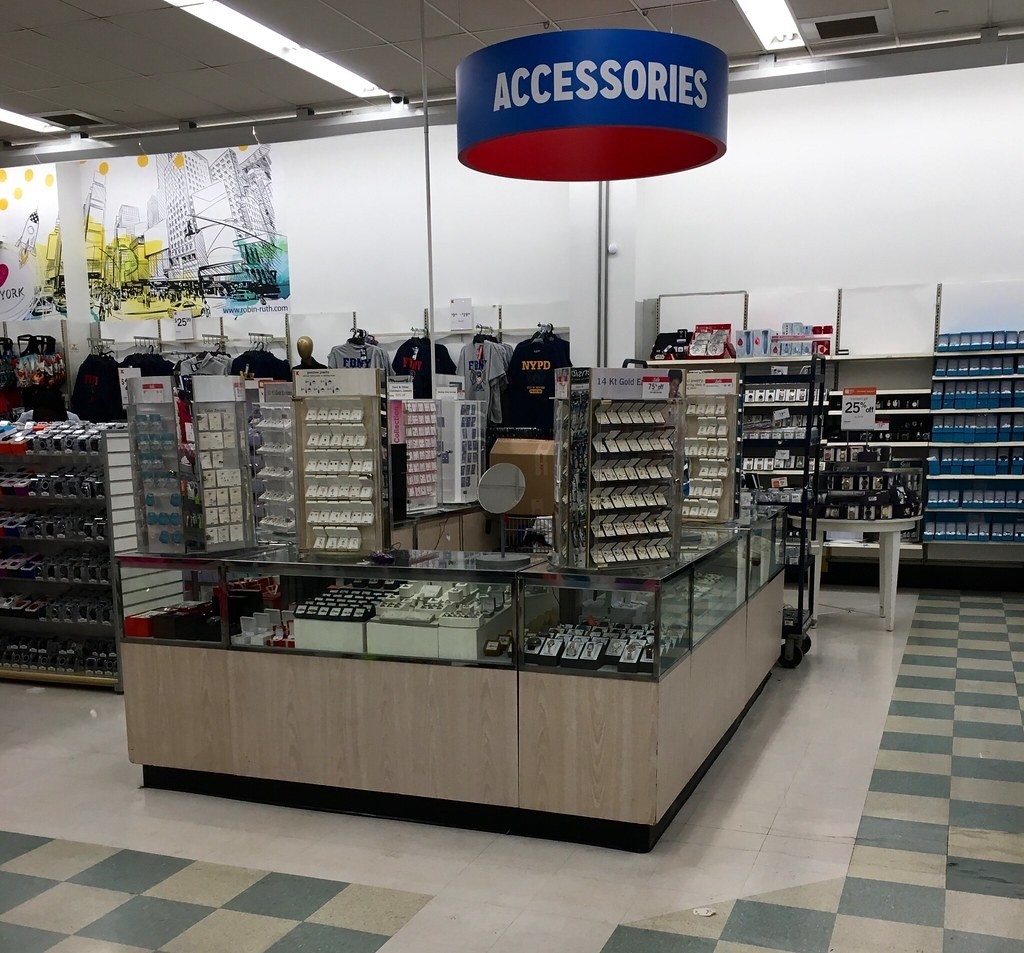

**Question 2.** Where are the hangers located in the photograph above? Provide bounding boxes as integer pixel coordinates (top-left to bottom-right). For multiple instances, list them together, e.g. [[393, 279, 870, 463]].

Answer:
[[349, 322, 557, 344], [88, 340, 273, 357]]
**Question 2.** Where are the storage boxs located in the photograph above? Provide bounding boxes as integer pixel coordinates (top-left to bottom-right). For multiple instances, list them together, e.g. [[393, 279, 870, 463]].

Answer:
[[991, 357, 1002, 375], [934, 358, 947, 376], [969, 358, 980, 376], [735, 322, 813, 358], [923, 379, 1024, 542], [1003, 356, 1014, 375], [959, 332, 971, 351], [970, 332, 981, 350], [980, 358, 991, 376], [981, 331, 993, 350], [1016, 356, 1024, 374], [937, 334, 950, 352], [1017, 331, 1024, 349], [122, 392, 730, 674], [1005, 331, 1018, 349], [947, 359, 958, 376], [993, 331, 1006, 350], [948, 334, 960, 352], [957, 359, 969, 376]]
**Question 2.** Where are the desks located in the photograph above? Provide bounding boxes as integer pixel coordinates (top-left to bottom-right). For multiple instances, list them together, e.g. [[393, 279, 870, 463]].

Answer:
[[787, 514, 923, 633]]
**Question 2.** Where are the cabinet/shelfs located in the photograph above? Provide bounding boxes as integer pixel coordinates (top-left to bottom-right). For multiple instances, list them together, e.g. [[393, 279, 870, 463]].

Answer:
[[0, 430, 186, 695], [823, 352, 933, 566], [291, 366, 384, 557], [254, 381, 295, 533], [645, 355, 830, 563], [923, 351, 1024, 570]]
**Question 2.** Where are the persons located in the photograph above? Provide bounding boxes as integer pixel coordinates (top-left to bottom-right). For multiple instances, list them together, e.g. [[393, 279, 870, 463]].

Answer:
[[667, 370, 683, 398], [291, 335, 327, 371]]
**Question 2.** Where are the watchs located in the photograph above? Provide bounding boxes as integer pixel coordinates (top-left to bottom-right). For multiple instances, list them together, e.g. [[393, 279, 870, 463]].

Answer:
[[745, 389, 806, 401], [0, 421, 118, 676], [819, 399, 929, 520], [489, 616, 686, 660]]
